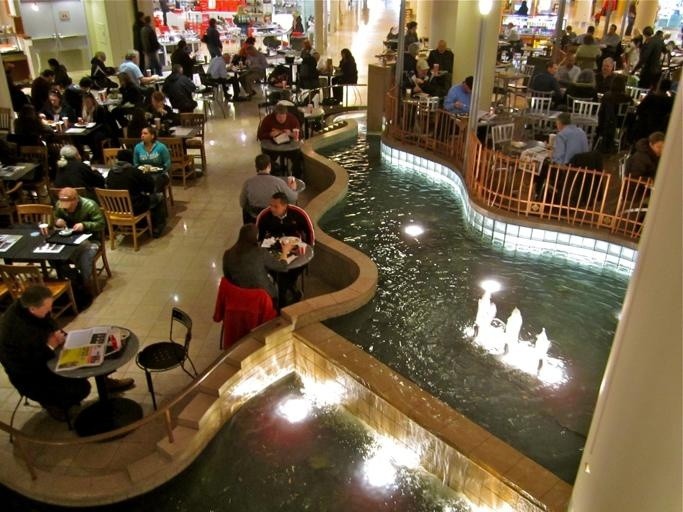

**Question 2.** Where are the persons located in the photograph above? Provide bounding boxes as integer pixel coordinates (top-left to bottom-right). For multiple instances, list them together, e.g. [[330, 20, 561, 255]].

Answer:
[[254, 191, 313, 302], [47, 186, 105, 307], [221, 223, 292, 307], [401, 19, 683, 211], [0, 286, 136, 421], [0, 1, 359, 238]]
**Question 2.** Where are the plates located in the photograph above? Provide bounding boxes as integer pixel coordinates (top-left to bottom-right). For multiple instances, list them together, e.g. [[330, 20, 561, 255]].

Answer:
[[58, 229, 72, 237], [511, 142, 526, 149]]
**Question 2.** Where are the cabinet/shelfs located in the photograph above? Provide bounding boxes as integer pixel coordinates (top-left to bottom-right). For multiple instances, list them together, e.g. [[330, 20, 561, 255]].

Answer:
[[18, 0, 91, 39], [499, 0, 564, 45]]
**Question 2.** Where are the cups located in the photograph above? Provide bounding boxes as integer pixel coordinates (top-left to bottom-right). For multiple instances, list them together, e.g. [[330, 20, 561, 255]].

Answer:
[[433, 64, 439, 76], [145, 69, 151, 78], [287, 176, 295, 184], [281, 81, 296, 92], [225, 61, 246, 73], [54, 117, 68, 136], [149, 118, 163, 132], [292, 129, 299, 142], [38, 224, 48, 236], [298, 103, 312, 116], [297, 244, 306, 255], [489, 104, 504, 117], [406, 89, 411, 98], [97, 87, 118, 103], [548, 134, 554, 144]]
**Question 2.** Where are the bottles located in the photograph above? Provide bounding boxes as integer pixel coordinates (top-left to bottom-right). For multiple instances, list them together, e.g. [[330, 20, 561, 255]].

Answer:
[[267, 236, 299, 259], [514, 18, 557, 36]]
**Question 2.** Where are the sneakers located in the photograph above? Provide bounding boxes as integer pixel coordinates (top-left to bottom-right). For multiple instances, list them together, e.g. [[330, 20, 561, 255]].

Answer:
[[290, 284, 303, 299], [244, 96, 251, 101], [247, 90, 257, 97], [107, 377, 136, 393], [224, 94, 232, 99], [233, 95, 245, 102], [41, 403, 72, 422], [147, 227, 160, 238]]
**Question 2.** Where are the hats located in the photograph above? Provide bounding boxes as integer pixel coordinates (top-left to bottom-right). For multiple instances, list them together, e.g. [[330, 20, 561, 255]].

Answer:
[[415, 58, 431, 72], [55, 186, 79, 210]]
